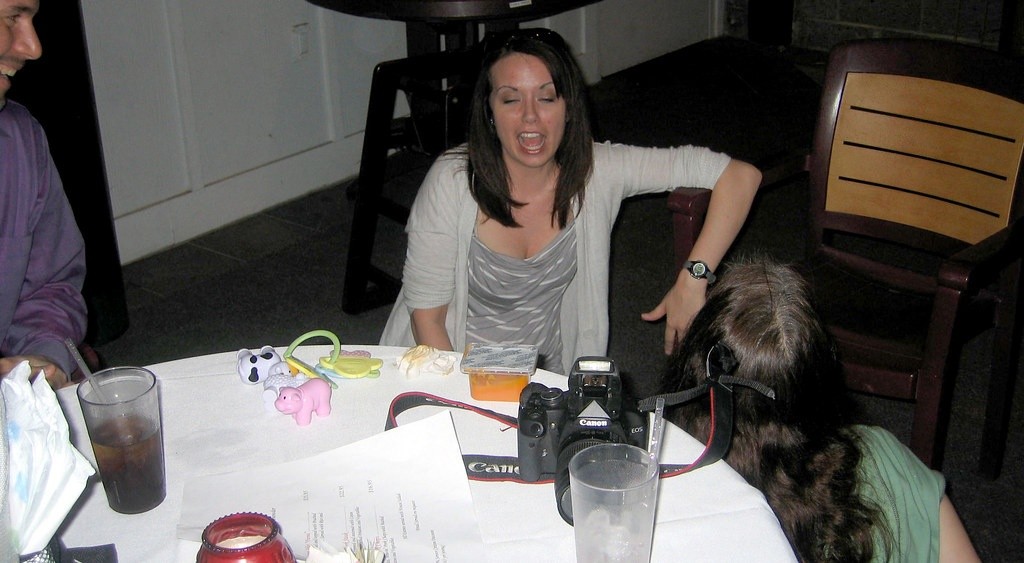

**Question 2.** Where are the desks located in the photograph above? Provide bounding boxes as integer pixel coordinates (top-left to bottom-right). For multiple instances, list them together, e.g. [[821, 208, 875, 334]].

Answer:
[[0, 346, 799, 563], [303, 0, 604, 315]]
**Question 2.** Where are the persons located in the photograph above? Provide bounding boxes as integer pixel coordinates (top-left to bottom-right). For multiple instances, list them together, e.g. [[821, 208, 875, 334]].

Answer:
[[375, 25, 764, 374], [661, 259, 982, 563], [1, 0, 100, 391]]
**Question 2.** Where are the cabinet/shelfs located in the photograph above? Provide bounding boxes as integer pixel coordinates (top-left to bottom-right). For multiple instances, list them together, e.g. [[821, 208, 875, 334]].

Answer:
[[82, 0, 723, 268]]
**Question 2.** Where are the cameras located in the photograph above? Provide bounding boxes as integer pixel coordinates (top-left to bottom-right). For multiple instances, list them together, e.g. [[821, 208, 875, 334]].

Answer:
[[517, 356, 649, 526]]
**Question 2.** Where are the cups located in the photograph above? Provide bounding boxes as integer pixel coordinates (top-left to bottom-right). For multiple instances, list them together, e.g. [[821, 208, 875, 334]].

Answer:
[[569, 443, 660, 563], [77, 366, 165, 513]]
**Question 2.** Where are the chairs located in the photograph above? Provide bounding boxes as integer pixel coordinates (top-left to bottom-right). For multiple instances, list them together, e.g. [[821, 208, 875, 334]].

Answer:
[[667, 39, 1024, 469]]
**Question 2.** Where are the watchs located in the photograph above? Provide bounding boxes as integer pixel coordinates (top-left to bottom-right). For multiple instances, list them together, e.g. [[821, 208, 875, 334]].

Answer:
[[679, 259, 718, 283]]
